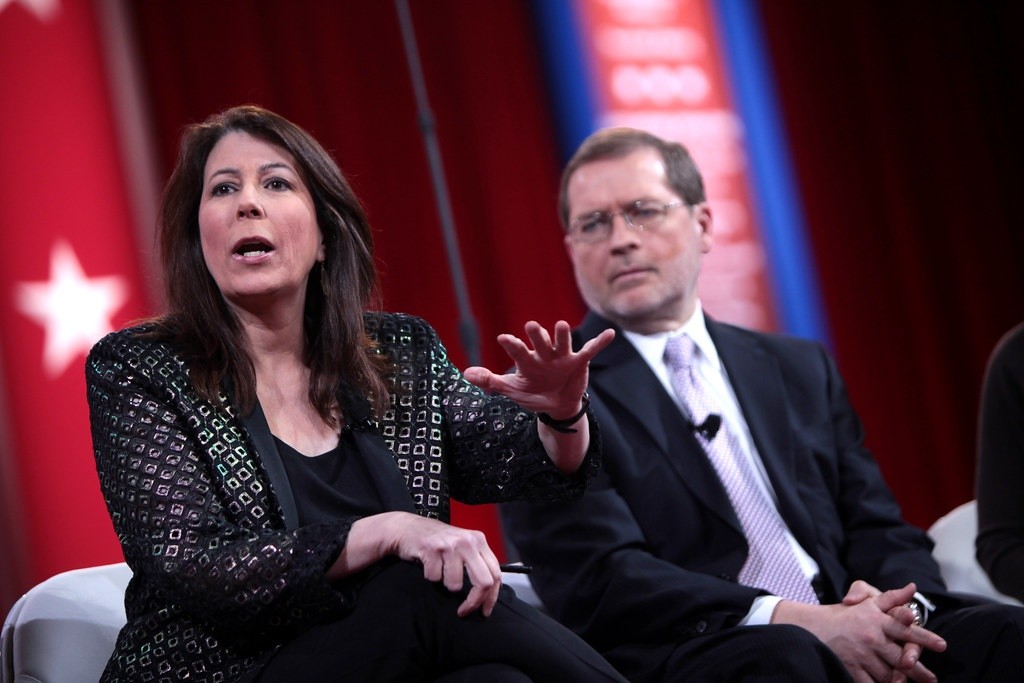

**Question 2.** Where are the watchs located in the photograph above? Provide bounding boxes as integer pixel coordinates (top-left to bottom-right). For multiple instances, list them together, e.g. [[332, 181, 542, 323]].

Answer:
[[907, 602, 925, 628], [535, 390, 591, 434]]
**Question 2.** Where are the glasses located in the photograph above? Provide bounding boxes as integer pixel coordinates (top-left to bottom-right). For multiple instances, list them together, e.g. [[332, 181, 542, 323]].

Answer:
[[567, 199, 692, 245]]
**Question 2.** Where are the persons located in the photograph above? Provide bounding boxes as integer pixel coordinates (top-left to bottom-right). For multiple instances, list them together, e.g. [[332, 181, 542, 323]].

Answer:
[[498, 125, 1024, 683], [973, 324, 1024, 603], [84, 104, 629, 682]]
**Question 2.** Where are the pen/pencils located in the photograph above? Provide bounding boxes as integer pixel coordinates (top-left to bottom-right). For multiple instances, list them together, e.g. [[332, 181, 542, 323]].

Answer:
[[414, 560, 534, 574]]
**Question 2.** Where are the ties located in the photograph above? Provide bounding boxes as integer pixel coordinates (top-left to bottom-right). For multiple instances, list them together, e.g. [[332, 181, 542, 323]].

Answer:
[[665, 334, 824, 610]]
